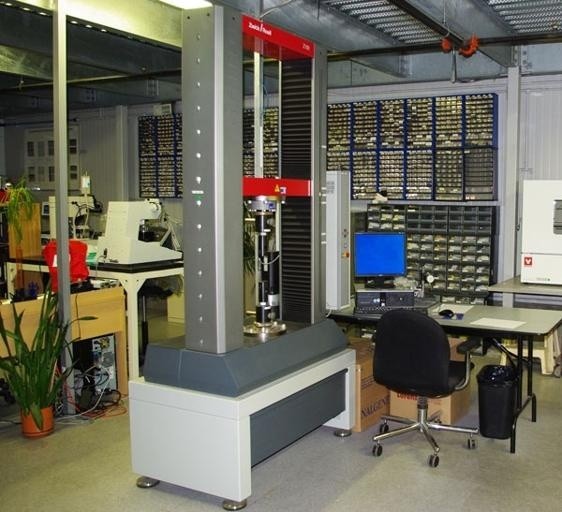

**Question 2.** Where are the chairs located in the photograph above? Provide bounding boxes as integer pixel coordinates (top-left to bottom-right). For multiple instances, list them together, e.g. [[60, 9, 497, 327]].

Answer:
[[372, 309, 479, 467]]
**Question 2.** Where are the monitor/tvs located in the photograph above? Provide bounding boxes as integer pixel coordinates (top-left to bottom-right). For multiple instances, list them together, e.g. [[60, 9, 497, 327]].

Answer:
[[352, 231, 407, 288]]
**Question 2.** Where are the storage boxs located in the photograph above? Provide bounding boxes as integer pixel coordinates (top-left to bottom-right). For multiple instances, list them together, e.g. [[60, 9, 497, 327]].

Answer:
[[348, 337, 389, 433], [390, 338, 470, 426]]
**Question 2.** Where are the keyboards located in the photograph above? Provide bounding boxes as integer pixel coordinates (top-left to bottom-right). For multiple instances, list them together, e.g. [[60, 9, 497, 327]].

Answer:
[[352, 306, 416, 319]]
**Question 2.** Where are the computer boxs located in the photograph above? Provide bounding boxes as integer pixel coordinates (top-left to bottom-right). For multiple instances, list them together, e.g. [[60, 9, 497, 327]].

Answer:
[[353, 288, 416, 306], [73, 335, 118, 395]]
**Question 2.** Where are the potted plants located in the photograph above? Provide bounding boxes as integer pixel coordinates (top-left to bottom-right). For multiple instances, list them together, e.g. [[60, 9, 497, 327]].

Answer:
[[0, 175, 36, 289], [0, 274, 98, 438]]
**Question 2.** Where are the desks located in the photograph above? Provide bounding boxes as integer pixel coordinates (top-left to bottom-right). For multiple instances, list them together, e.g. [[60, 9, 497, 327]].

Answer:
[[326, 303, 562, 453], [488, 275, 562, 308]]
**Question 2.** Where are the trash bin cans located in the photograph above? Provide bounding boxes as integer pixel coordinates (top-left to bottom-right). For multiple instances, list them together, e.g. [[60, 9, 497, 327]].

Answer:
[[478, 364, 523, 439]]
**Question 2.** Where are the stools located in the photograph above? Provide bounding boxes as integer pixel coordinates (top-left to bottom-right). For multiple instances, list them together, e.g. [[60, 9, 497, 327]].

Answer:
[[500, 330, 560, 375]]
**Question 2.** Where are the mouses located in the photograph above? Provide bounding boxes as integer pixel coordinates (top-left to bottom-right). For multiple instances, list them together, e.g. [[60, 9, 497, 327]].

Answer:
[[439, 309, 454, 317]]
